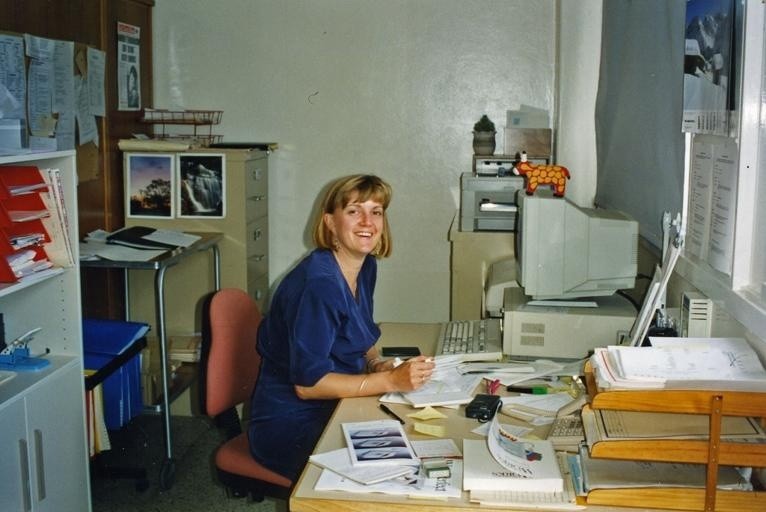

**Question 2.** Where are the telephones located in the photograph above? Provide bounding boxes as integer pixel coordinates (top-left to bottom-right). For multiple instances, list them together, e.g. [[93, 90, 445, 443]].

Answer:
[[546, 395, 591, 453]]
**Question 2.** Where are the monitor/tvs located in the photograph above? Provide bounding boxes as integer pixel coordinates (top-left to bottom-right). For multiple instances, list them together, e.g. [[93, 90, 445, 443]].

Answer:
[[516, 190, 640, 308]]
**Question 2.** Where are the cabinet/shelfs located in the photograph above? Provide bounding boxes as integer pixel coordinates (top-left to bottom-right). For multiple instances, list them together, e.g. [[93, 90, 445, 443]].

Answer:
[[0, 146, 95, 511], [577, 354, 766, 512], [119, 146, 268, 418]]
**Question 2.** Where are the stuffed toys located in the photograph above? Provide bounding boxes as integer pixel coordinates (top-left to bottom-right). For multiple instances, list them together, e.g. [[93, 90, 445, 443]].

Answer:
[[513, 148, 573, 199]]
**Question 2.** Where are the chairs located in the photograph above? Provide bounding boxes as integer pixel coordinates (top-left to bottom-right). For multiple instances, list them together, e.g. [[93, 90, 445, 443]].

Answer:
[[194, 289, 299, 510]]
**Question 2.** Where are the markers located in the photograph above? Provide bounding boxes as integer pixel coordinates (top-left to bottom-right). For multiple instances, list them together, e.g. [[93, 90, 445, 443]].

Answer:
[[507, 386, 548, 394]]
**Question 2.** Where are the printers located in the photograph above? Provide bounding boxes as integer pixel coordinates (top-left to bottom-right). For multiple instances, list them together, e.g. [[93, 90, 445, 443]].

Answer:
[[460, 172, 551, 232]]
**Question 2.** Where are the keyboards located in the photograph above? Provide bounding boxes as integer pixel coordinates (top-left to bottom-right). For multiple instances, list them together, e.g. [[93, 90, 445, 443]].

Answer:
[[434, 319, 502, 362]]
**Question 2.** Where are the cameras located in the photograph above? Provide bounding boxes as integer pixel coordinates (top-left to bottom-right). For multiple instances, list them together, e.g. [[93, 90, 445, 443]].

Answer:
[[465, 394, 500, 420]]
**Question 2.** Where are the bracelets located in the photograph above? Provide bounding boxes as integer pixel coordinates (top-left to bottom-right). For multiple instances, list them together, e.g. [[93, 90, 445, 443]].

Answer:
[[365, 357, 380, 373], [358, 374, 370, 396]]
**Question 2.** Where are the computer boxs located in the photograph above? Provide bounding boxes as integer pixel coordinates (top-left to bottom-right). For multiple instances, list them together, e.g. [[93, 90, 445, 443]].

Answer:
[[501, 287, 639, 360]]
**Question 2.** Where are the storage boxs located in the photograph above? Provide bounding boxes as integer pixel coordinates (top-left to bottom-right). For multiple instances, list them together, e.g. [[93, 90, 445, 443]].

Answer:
[[140, 335, 175, 406], [503, 125, 553, 156]]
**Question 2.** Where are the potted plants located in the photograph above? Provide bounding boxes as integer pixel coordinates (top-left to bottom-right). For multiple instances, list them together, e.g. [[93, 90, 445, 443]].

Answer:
[[470, 114, 499, 156]]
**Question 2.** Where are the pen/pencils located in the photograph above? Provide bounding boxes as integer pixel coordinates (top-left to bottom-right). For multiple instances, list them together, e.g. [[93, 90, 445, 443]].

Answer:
[[422, 358, 436, 362], [379, 404, 405, 424]]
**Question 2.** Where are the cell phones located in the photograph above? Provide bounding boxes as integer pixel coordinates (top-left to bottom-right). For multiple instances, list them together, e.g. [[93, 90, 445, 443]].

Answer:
[[382, 347, 420, 356]]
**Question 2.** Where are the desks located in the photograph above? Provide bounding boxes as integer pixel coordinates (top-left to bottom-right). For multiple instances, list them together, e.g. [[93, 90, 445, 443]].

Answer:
[[285, 317, 766, 512], [445, 210, 517, 321], [79, 231, 224, 459]]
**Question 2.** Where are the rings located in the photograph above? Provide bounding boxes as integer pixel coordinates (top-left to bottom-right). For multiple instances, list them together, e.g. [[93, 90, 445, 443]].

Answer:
[[421, 373, 426, 384]]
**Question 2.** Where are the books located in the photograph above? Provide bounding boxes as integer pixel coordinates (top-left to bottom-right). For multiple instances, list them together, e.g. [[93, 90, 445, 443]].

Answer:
[[6, 180, 49, 197], [167, 332, 204, 363], [8, 208, 53, 225], [83, 369, 113, 458], [104, 224, 178, 251]]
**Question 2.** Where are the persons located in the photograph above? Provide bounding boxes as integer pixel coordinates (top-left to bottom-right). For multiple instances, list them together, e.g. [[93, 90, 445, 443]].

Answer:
[[243, 173, 438, 483], [126, 64, 140, 106]]
